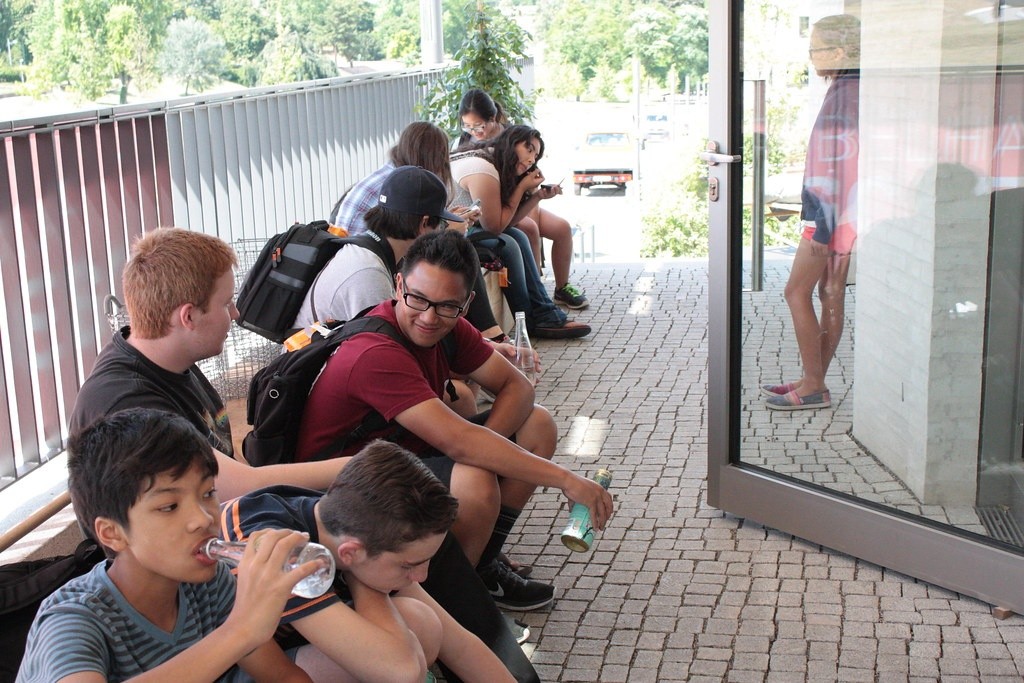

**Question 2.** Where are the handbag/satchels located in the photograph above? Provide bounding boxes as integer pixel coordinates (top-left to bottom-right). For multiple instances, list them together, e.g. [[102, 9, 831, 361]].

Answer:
[[469, 229, 516, 334]]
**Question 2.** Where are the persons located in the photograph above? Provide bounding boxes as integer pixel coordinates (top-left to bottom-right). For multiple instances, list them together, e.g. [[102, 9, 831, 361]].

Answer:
[[247, 229, 613, 610], [13, 408, 335, 683], [67, 226, 532, 643], [217, 439, 519, 683], [234, 88, 590, 420], [762, 14, 862, 411]]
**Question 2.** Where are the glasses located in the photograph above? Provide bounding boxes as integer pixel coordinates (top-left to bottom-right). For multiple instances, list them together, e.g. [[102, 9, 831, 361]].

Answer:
[[461, 119, 489, 133], [808, 47, 836, 57], [402, 278, 470, 318]]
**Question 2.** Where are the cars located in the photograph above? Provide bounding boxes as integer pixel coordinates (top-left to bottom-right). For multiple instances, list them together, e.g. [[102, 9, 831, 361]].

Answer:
[[647, 112, 671, 135]]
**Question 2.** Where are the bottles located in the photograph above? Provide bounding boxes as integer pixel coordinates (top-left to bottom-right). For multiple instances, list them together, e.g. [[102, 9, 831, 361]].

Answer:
[[561, 468, 612, 552], [199, 537, 336, 599], [512, 311, 536, 389]]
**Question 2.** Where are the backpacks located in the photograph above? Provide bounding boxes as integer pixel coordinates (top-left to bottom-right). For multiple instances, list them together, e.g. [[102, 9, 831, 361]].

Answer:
[[241, 306, 455, 476], [235, 220, 389, 346], [0, 540, 110, 683]]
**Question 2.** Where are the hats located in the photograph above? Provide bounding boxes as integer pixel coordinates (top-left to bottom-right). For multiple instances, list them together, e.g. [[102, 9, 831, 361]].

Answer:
[[377, 165, 466, 222]]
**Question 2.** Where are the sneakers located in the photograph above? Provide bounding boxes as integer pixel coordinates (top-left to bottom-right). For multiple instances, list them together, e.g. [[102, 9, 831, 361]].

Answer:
[[554, 282, 589, 309], [478, 558, 557, 610]]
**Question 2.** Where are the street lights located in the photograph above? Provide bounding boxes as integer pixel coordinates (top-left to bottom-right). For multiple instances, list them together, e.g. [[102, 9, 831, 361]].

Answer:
[[20, 58, 24, 84], [7, 38, 17, 65]]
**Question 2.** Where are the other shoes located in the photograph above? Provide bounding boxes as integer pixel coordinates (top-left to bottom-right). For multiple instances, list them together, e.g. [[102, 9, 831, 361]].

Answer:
[[765, 390, 831, 410], [762, 382, 794, 395], [511, 561, 532, 577], [536, 320, 592, 338]]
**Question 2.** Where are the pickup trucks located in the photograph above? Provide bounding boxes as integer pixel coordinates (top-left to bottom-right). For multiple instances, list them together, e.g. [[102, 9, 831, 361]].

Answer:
[[574, 131, 636, 195]]
